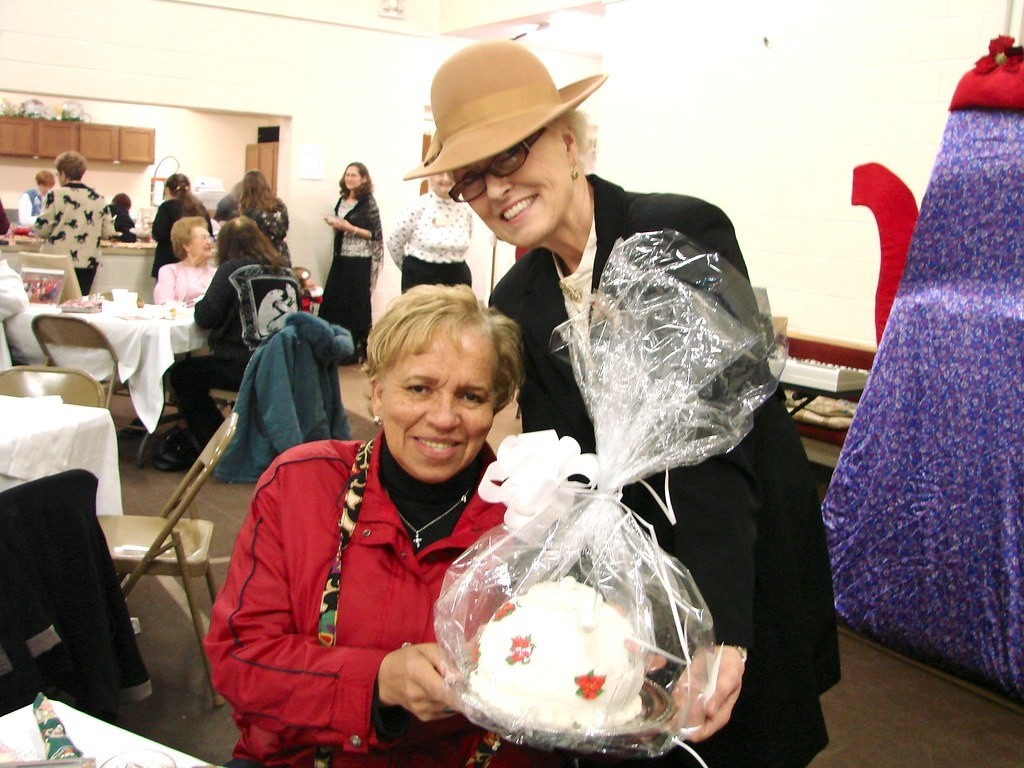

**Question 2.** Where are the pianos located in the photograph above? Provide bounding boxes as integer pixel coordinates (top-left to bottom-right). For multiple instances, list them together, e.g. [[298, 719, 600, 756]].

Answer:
[[769, 355, 872, 418]]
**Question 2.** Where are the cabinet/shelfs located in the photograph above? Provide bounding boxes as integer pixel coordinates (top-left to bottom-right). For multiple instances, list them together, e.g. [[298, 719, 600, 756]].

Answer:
[[0, 115, 156, 164]]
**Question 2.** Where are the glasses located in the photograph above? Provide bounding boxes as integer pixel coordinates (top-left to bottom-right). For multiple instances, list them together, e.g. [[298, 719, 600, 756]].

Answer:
[[448, 126, 548, 204]]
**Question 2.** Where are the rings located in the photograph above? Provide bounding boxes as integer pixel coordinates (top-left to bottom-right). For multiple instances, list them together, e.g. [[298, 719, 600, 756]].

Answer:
[[444, 706, 454, 713]]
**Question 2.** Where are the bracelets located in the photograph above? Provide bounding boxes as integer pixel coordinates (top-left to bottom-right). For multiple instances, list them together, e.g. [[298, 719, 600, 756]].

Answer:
[[733, 644, 748, 662]]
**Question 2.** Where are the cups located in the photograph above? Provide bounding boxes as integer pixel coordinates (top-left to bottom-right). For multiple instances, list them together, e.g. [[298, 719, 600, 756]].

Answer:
[[112, 288, 187, 321]]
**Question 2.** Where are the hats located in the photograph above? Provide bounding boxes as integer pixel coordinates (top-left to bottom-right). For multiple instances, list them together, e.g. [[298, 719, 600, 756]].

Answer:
[[403, 39, 608, 181]]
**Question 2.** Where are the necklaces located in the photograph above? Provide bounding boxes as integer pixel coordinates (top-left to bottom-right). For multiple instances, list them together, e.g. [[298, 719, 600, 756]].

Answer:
[[398, 490, 470, 549]]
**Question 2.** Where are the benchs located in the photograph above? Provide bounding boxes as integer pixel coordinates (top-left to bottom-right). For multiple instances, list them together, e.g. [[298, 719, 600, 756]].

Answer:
[[789, 333, 875, 466]]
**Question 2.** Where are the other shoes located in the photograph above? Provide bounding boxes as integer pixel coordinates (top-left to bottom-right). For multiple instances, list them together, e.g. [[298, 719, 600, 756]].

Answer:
[[341, 345, 370, 366]]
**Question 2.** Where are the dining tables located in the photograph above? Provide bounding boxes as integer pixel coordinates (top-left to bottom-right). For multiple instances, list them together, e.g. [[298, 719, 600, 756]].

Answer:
[[3, 300, 211, 465]]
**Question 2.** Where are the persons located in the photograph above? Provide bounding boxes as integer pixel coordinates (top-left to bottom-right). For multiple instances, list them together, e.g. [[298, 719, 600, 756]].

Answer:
[[34, 151, 113, 296], [242, 170, 291, 262], [108, 194, 137, 241], [203, 283, 527, 768], [214, 181, 243, 226], [16, 170, 55, 227], [150, 174, 213, 279], [294, 267, 323, 312], [0, 199, 10, 236], [401, 42, 841, 767], [387, 170, 473, 292], [318, 162, 382, 365], [25, 280, 58, 299], [154, 216, 216, 306], [154, 217, 302, 473]]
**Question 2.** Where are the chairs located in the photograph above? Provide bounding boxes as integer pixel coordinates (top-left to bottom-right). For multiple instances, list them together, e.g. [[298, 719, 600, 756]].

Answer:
[[0, 366, 107, 408], [95, 412, 239, 704], [31, 313, 148, 432]]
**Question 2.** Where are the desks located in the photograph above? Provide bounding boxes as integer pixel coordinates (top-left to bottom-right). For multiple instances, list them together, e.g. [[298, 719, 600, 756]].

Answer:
[[0, 394, 123, 677]]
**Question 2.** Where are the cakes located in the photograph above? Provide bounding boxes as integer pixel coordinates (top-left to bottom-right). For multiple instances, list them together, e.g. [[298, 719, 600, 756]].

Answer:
[[469, 575, 643, 729]]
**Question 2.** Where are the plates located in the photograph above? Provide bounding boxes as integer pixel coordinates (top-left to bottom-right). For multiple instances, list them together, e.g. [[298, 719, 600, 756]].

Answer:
[[456, 659, 674, 742]]
